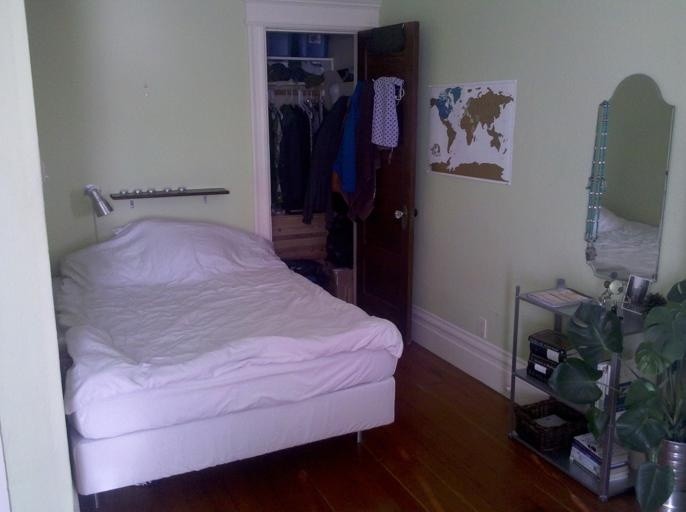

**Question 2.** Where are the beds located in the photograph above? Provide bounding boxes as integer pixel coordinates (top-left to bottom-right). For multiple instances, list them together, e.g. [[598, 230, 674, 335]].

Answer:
[[591, 206, 660, 277], [52, 215, 404, 510]]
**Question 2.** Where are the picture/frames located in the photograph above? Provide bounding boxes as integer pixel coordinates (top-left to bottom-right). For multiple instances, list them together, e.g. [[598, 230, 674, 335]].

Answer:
[[425, 79, 517, 185]]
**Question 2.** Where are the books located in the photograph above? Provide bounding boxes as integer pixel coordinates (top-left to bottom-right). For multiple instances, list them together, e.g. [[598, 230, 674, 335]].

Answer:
[[570, 430, 631, 482], [526, 327, 585, 385], [526, 288, 593, 308]]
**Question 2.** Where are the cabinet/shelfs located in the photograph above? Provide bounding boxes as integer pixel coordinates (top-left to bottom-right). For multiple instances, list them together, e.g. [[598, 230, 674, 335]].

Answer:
[[247, 2, 420, 347], [505, 277, 661, 505]]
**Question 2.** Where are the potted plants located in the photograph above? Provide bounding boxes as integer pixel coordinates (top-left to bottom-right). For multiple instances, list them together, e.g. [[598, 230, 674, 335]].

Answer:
[[549, 279, 686, 512]]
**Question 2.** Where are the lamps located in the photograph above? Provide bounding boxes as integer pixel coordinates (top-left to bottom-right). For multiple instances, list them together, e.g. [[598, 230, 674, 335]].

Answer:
[[83, 183, 113, 218]]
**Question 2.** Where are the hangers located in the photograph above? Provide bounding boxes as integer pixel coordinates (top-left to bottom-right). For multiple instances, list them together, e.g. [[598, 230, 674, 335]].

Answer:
[[267, 80, 329, 118]]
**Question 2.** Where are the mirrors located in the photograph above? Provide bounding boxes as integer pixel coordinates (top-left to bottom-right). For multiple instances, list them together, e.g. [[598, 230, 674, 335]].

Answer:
[[585, 73, 675, 284]]
